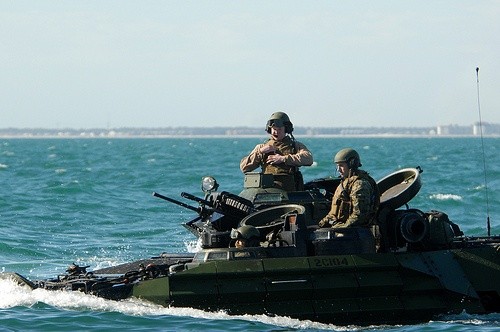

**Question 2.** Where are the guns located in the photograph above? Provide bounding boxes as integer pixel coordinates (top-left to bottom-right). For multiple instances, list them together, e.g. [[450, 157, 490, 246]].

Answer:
[[181, 191, 254, 220], [152, 192, 210, 215]]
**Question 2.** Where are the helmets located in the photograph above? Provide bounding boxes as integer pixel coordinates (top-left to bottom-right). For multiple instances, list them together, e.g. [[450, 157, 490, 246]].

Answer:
[[266, 112, 293, 134], [335, 148, 362, 167]]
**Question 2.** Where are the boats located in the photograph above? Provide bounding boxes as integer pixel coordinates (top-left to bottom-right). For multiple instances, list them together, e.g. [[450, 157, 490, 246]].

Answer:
[[0, 165, 500, 328]]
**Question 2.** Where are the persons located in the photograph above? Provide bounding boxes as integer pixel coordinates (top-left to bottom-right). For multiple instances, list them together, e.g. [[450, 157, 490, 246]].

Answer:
[[240, 111, 313, 189], [319, 148, 375, 232]]
